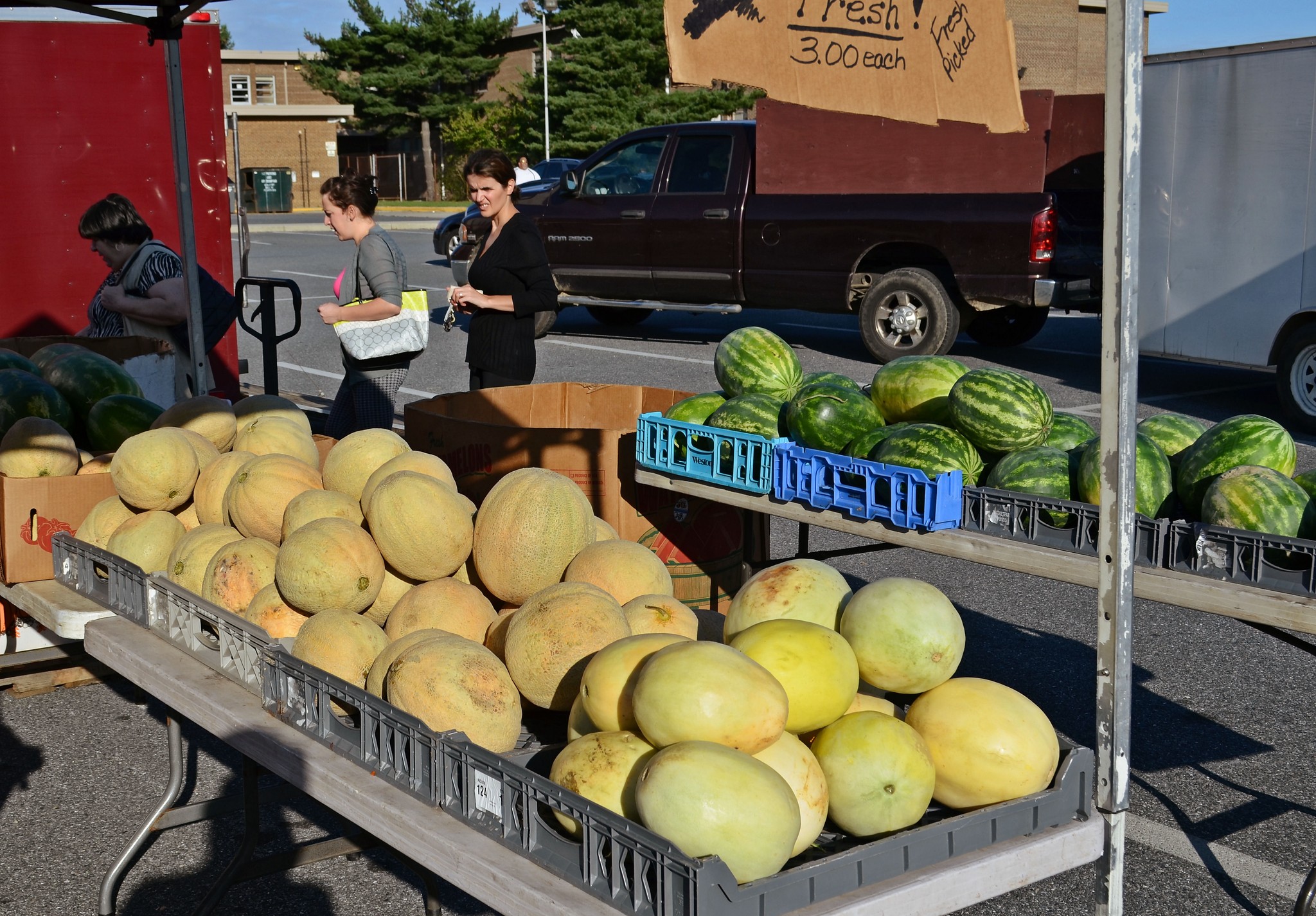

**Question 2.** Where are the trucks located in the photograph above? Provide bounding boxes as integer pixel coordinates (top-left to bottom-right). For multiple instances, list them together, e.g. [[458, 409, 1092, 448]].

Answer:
[[1144, 37, 1315, 444]]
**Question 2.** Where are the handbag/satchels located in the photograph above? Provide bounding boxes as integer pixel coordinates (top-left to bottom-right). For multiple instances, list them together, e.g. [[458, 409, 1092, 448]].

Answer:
[[330, 233, 430, 360], [113, 245, 240, 357]]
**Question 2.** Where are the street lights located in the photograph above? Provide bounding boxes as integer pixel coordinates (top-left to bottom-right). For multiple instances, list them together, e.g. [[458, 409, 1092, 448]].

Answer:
[[520, 0, 559, 162]]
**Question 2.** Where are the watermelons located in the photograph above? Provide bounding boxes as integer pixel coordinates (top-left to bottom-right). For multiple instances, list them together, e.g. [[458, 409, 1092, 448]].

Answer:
[[0, 343, 166, 452], [662, 326, 1316, 542]]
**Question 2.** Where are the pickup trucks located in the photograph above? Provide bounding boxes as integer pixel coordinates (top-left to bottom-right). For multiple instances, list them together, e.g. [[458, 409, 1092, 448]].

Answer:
[[433, 120, 1108, 363]]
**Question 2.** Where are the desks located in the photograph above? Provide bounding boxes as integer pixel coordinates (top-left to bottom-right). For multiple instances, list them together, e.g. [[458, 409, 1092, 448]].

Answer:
[[80, 618, 1104, 916], [0, 580, 118, 639], [633, 446, 1316, 916]]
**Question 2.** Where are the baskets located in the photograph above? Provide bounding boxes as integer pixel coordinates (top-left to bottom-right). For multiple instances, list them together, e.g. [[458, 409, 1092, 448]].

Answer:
[[51, 526, 1098, 916], [634, 405, 1316, 599]]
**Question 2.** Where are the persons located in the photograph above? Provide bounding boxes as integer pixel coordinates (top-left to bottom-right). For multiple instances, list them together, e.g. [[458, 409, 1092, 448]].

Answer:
[[316, 166, 410, 438], [445, 149, 557, 393], [72, 191, 215, 404]]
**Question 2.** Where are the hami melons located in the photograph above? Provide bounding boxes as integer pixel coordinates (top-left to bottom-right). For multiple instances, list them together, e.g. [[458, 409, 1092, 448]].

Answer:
[[77, 390, 1061, 888], [0, 417, 116, 541]]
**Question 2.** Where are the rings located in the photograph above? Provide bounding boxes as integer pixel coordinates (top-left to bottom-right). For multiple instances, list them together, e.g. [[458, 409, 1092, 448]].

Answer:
[[459, 292, 463, 297]]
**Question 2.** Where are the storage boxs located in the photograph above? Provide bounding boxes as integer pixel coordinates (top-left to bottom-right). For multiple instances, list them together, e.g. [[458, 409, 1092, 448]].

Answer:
[[0, 329, 1316, 916]]
